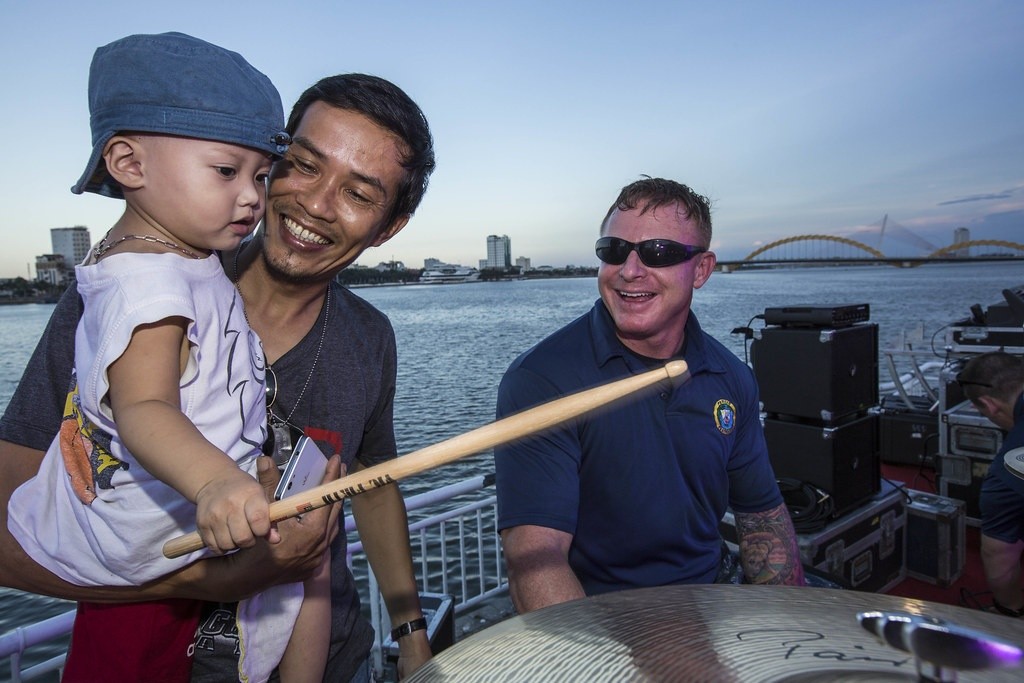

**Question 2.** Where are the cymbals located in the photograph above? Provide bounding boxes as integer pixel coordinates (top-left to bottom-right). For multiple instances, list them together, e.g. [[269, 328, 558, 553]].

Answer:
[[1003, 447, 1024, 481], [396, 580, 1024, 683]]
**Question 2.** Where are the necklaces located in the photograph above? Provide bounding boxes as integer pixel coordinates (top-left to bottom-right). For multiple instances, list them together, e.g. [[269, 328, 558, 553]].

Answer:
[[234, 242, 332, 469], [93, 226, 206, 259]]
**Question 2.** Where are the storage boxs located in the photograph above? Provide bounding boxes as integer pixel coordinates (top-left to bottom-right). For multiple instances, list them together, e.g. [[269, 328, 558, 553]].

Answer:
[[941, 399, 1008, 463], [879, 407, 939, 468], [945, 317, 1024, 354], [906, 487, 969, 589], [717, 477, 910, 596]]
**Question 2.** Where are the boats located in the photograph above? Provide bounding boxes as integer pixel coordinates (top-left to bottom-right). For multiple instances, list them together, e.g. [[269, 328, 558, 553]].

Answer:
[[419, 268, 481, 285]]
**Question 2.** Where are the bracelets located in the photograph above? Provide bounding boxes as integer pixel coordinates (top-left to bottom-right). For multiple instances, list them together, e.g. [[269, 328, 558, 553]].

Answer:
[[391, 618, 428, 642]]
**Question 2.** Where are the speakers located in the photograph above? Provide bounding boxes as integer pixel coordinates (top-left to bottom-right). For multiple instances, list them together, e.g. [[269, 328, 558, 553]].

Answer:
[[749, 321, 881, 519]]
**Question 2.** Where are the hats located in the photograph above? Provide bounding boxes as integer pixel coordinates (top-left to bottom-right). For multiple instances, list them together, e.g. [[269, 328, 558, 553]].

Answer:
[[70, 32, 293, 195]]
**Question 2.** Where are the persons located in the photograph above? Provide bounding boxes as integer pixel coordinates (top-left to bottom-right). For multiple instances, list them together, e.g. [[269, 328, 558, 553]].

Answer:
[[7, 30, 332, 683], [959, 353, 1023, 618], [1, 75, 435, 682], [496, 181, 808, 616]]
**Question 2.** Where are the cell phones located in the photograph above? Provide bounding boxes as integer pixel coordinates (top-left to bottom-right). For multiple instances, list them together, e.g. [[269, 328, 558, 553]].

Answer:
[[274, 435, 328, 501]]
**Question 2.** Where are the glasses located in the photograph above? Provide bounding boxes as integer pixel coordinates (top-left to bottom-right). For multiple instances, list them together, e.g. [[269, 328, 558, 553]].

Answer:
[[956, 369, 994, 388], [594, 236, 706, 268], [262, 351, 278, 456]]
